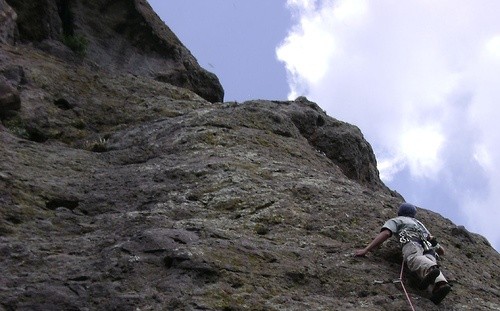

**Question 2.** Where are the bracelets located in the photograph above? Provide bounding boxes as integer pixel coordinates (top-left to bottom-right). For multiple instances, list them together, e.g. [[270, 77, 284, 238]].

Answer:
[[364, 247, 370, 252]]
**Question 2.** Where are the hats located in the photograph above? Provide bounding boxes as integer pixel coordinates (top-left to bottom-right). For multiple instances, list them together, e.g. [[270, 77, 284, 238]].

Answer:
[[398, 203, 417, 217]]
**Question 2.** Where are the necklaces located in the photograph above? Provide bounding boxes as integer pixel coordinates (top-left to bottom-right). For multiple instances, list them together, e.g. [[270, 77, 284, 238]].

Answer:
[[355, 204, 449, 305]]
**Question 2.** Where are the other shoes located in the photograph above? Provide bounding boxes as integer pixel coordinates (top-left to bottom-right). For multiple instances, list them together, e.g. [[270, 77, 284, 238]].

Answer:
[[418, 269, 440, 289], [429, 284, 451, 305]]
